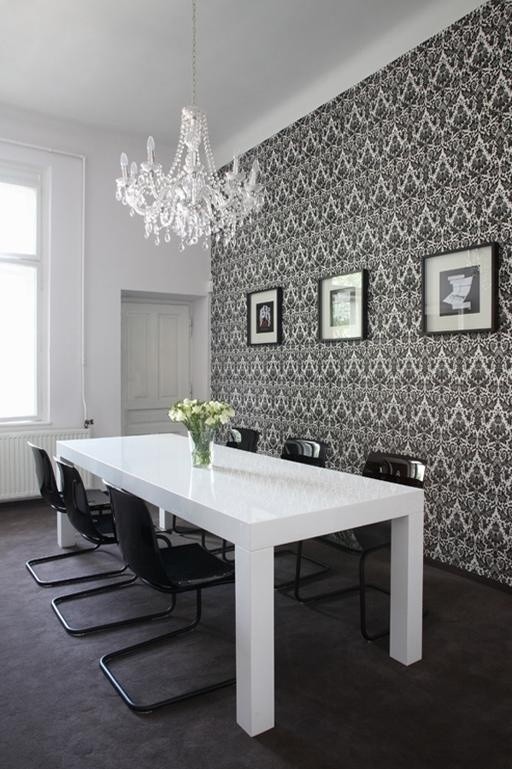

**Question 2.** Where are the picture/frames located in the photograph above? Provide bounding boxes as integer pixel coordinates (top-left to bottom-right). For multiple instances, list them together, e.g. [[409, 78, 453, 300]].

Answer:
[[247, 287, 283, 345], [420, 240, 499, 336], [316, 269, 365, 342]]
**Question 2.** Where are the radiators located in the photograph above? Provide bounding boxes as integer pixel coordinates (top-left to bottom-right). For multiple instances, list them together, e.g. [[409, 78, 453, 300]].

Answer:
[[1, 426, 96, 500]]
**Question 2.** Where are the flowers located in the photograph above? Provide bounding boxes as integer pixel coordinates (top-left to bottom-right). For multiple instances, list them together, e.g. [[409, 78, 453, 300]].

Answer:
[[167, 395, 236, 432]]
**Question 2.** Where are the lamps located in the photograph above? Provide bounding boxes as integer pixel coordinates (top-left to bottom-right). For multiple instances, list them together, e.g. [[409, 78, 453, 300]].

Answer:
[[112, 2, 269, 256]]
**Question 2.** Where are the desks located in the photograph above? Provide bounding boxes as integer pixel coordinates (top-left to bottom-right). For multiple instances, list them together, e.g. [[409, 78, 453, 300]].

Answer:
[[55, 433, 427, 741]]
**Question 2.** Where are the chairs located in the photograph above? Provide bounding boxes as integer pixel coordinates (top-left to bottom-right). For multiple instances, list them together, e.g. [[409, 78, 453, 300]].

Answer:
[[100, 478, 234, 714], [273, 436, 378, 605], [20, 437, 125, 585], [296, 453, 434, 646], [200, 425, 292, 576], [49, 455, 178, 635]]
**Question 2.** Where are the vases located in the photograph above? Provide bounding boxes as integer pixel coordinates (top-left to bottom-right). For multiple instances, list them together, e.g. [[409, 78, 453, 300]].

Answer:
[[187, 431, 211, 468]]
